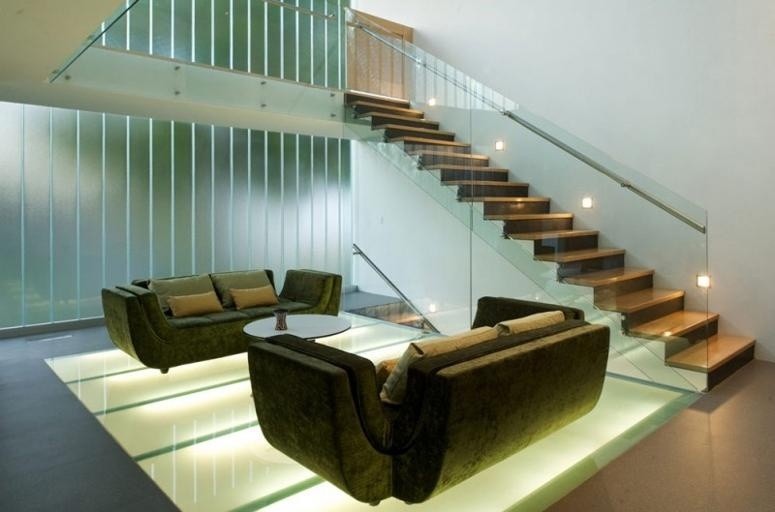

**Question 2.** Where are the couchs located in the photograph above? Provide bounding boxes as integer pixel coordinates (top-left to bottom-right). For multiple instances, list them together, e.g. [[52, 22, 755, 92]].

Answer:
[[247, 295, 610, 507], [102, 268, 342, 374]]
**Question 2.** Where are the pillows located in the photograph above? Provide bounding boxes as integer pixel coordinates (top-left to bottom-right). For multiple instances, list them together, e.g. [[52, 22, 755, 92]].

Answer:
[[166, 289, 225, 317], [380, 326, 498, 405], [492, 309, 566, 336], [211, 269, 281, 308], [229, 284, 280, 310], [376, 358, 400, 392], [146, 273, 214, 313]]
